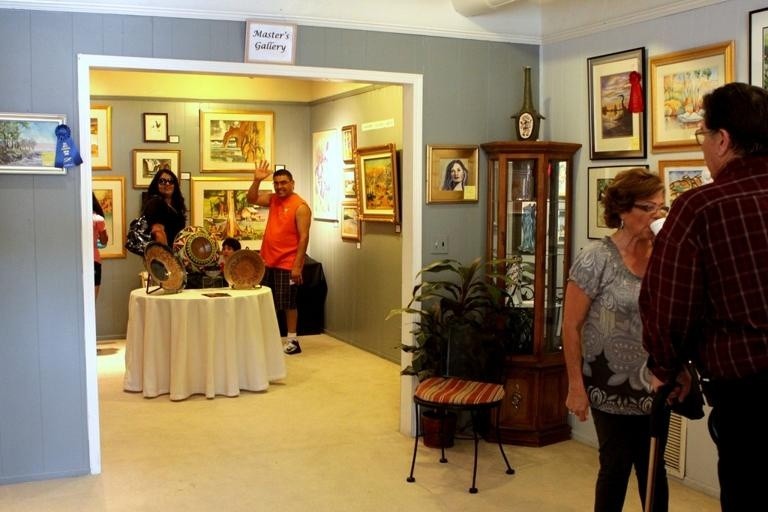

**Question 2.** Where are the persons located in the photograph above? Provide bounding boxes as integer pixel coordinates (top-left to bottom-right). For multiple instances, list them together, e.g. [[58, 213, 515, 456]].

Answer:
[[144, 168, 186, 281], [246, 160, 311, 354], [93, 193, 108, 300], [441, 160, 468, 192], [638, 82, 766, 512], [218, 238, 241, 274], [561, 168, 669, 512]]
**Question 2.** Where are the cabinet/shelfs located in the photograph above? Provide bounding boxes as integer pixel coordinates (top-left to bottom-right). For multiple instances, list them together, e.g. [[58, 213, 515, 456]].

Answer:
[[480, 138, 577, 447]]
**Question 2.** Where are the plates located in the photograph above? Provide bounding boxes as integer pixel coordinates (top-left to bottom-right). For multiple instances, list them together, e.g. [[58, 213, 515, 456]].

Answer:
[[223, 249, 265, 290], [172, 225, 221, 274], [144, 241, 187, 292]]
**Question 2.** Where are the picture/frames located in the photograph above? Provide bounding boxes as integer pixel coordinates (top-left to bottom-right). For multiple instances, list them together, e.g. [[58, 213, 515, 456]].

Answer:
[[142, 111, 170, 144], [425, 143, 481, 206], [585, 47, 649, 161], [585, 165, 650, 239], [646, 39, 735, 156], [198, 109, 277, 176], [0, 112, 69, 178], [129, 147, 182, 190], [747, 6, 768, 93], [309, 128, 343, 224], [88, 106, 113, 172], [341, 124, 402, 241], [187, 176, 274, 253], [655, 160, 717, 221], [244, 17, 299, 67], [88, 176, 128, 260]]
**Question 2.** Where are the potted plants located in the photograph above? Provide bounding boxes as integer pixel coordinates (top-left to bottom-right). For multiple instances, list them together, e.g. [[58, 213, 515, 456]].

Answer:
[[384, 254, 525, 450]]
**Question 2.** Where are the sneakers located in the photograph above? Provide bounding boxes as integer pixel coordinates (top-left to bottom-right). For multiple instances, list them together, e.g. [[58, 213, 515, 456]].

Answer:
[[285, 340, 301, 355]]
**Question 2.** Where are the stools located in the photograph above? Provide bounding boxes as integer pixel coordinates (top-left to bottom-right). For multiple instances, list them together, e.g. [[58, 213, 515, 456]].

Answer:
[[406, 376, 517, 495]]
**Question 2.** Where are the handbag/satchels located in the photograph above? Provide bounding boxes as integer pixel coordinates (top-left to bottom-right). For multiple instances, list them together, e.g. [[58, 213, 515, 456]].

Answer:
[[669, 358, 706, 421], [124, 216, 153, 257]]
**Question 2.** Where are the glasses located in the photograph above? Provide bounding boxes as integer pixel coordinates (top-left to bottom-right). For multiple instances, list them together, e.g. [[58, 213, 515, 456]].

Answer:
[[158, 178, 176, 185], [633, 203, 669, 216], [694, 128, 717, 143]]
